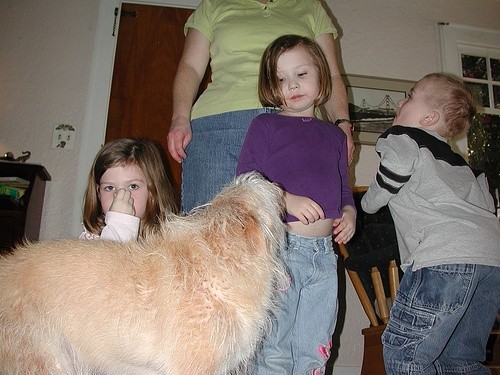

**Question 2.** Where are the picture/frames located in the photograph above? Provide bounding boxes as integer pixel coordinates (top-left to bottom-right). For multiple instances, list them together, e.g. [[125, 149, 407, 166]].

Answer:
[[341, 74, 419, 145]]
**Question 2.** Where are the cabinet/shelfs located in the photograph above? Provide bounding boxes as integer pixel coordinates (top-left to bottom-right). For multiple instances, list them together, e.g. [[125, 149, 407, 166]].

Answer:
[[0, 161, 52, 255], [361, 328, 500, 375]]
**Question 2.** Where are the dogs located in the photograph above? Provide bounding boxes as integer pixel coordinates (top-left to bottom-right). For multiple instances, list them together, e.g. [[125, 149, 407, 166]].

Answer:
[[0, 170, 292, 375]]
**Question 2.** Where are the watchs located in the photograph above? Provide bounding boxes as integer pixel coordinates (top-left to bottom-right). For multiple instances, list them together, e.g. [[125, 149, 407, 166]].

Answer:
[[332, 118, 356, 131]]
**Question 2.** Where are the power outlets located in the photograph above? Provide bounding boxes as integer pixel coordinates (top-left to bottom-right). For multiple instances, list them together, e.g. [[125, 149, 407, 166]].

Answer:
[[51, 125, 76, 151]]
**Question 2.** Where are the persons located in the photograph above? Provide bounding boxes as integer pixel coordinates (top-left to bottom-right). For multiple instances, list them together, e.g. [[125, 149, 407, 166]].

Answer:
[[167, 1, 355, 216], [361, 72, 500, 374], [237, 35, 357, 375], [80, 137, 179, 243]]
